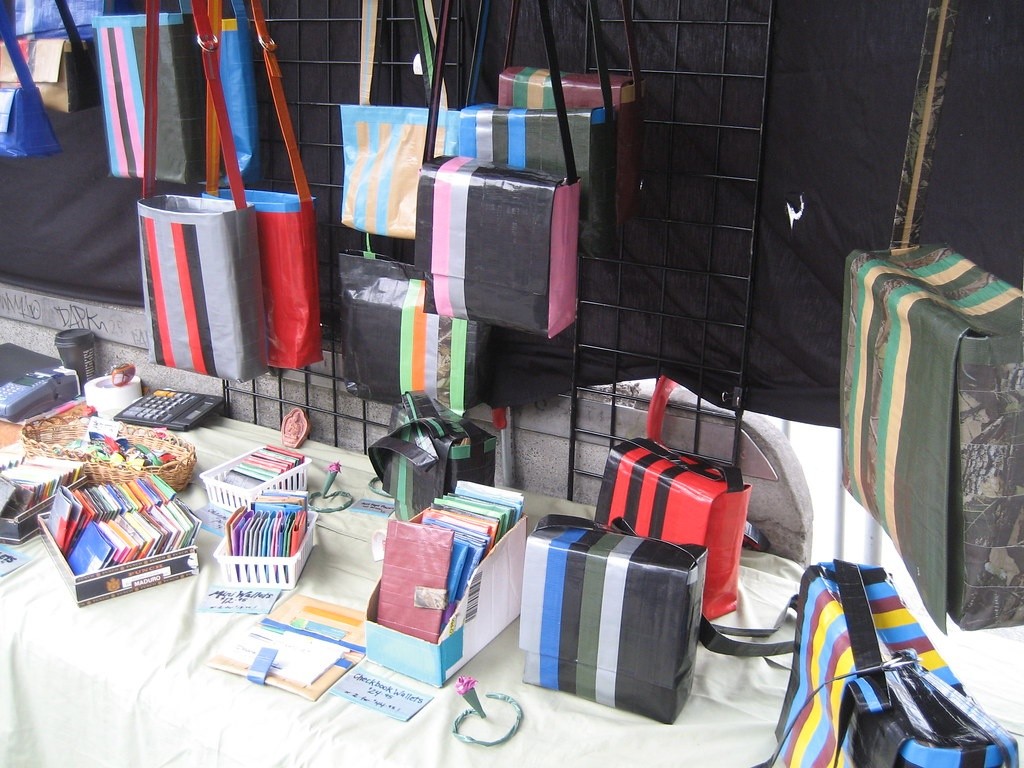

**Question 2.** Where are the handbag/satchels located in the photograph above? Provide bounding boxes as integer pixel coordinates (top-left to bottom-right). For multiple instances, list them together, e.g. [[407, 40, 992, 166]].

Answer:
[[92, 15, 259, 185], [367, 389, 496, 522], [203, 188, 324, 370], [593, 437, 753, 621], [0, 0, 101, 113], [499, 66, 647, 209], [841, 240, 1023, 636], [339, 104, 461, 241], [460, 104, 616, 259], [138, 195, 268, 383], [0, 0, 111, 43], [414, 154, 578, 338], [333, 248, 493, 418], [752, 558, 1020, 768], [519, 513, 801, 725], [0, 0, 63, 158]]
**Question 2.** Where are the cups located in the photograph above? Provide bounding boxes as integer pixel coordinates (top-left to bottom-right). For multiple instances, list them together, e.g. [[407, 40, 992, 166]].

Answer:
[[54, 329, 95, 385]]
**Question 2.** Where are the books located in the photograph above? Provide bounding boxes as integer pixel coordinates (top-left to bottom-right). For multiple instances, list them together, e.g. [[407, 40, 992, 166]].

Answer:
[[0, 455, 202, 576], [377, 480, 524, 644], [217, 445, 306, 508], [204, 593, 367, 702]]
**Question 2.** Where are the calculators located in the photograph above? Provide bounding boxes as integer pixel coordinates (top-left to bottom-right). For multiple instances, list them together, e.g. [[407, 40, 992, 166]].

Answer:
[[118, 390, 224, 432]]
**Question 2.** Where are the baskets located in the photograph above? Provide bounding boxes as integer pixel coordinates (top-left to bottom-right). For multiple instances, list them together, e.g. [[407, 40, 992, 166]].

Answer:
[[21, 416, 196, 491]]
